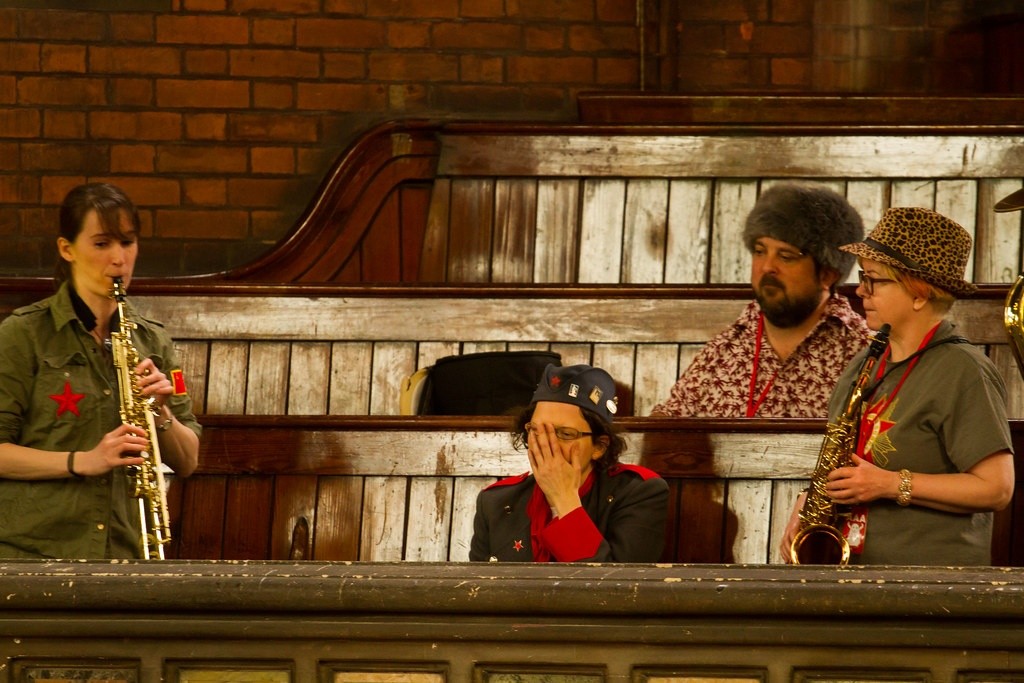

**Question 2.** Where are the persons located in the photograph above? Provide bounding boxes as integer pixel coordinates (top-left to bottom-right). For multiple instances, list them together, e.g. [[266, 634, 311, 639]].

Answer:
[[771, 206, 1017, 566], [649, 180, 879, 421], [467, 362, 669, 564], [0, 183, 199, 562]]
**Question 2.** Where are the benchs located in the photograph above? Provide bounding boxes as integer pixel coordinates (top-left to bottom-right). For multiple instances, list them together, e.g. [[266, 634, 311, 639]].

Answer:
[[0, 95, 1024, 568]]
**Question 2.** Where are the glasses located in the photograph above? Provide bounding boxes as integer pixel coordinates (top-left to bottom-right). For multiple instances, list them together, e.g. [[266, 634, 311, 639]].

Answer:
[[525, 422, 593, 440], [858, 270, 901, 295]]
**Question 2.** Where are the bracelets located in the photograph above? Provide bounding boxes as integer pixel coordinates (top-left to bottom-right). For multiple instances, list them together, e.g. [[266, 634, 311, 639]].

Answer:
[[896, 468, 912, 506], [60, 451, 86, 476]]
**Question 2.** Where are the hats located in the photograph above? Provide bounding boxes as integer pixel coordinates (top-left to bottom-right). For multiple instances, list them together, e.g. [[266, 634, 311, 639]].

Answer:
[[839, 207, 977, 296], [742, 184, 864, 287], [532, 364, 618, 424]]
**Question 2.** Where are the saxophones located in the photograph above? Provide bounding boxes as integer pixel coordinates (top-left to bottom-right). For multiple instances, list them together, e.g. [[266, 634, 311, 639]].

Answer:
[[789, 322, 893, 566], [110, 275, 175, 562]]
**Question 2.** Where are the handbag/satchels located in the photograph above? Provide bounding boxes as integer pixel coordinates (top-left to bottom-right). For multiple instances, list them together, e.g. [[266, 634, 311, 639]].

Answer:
[[399, 351, 563, 416]]
